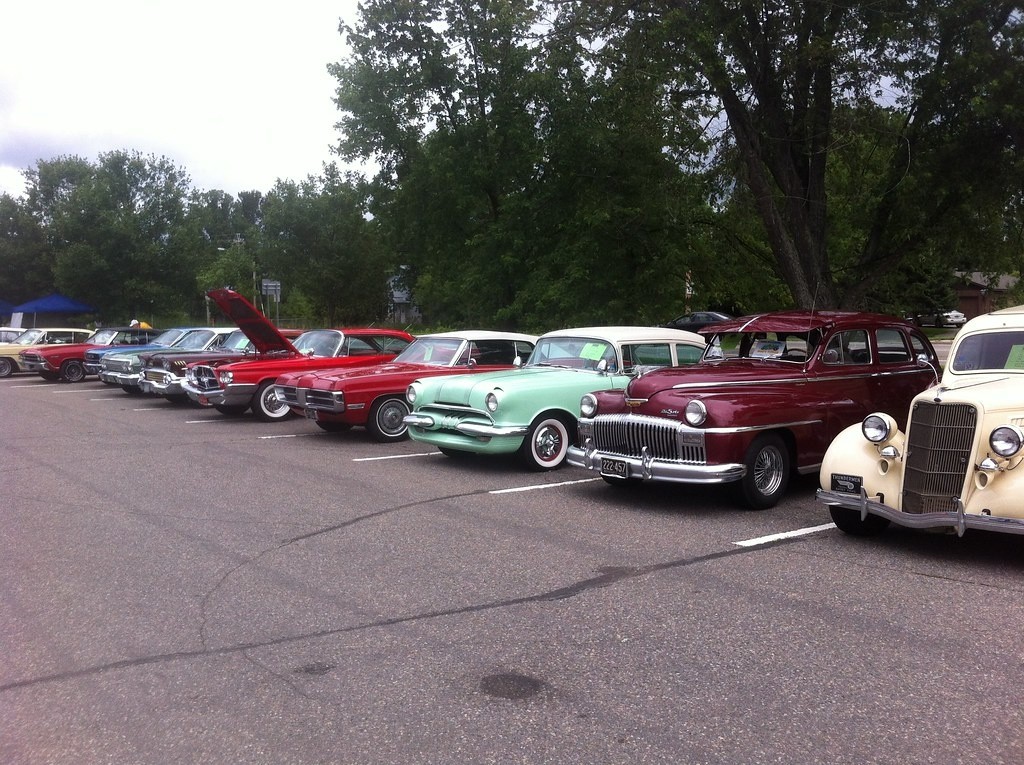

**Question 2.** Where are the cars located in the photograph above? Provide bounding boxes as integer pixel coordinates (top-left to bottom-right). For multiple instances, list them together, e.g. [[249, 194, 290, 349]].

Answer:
[[815, 304, 1024, 543], [659, 312, 736, 343], [914, 309, 967, 329], [183, 289, 480, 422], [567, 309, 943, 510], [276, 329, 576, 444], [0, 326, 308, 400], [402, 326, 711, 471]]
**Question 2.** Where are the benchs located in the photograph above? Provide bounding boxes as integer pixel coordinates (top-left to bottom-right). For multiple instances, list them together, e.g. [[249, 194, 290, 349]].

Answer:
[[851, 350, 915, 364], [475, 352, 532, 365]]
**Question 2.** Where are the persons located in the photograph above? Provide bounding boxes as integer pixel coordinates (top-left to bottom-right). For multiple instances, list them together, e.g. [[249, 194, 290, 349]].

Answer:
[[129, 319, 152, 329]]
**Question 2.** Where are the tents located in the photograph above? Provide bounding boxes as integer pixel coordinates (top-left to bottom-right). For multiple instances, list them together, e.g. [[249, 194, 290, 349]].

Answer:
[[0, 300, 18, 328], [11, 294, 100, 328]]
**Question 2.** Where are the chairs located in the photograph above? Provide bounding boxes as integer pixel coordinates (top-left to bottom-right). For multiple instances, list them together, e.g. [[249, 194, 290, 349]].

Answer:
[[327, 346, 405, 356], [120, 340, 128, 344], [825, 348, 849, 364]]
